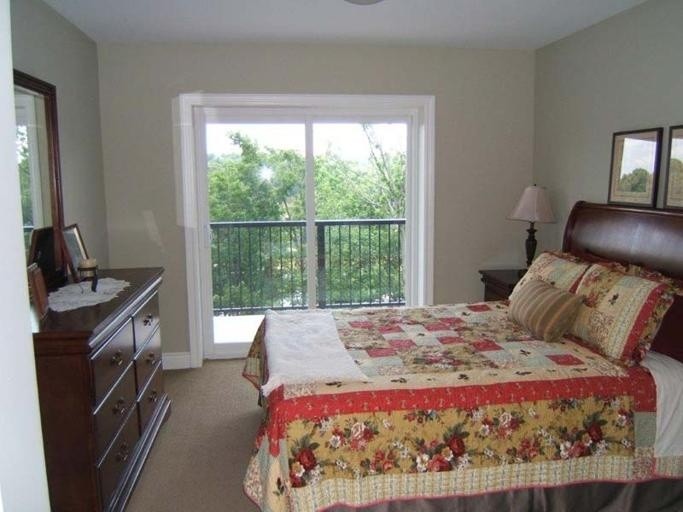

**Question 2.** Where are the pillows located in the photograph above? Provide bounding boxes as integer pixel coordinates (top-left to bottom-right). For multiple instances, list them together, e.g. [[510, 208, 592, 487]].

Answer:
[[503, 250, 680, 371]]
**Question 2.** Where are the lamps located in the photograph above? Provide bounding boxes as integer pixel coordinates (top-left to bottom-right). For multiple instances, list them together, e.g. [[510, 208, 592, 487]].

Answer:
[[505, 185, 559, 275]]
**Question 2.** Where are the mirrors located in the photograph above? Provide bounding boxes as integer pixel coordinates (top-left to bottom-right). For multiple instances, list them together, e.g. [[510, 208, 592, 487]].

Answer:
[[9, 69, 68, 290]]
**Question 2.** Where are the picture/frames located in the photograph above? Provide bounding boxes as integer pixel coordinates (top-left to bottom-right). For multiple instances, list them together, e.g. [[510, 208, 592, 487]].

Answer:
[[605, 125, 683, 213]]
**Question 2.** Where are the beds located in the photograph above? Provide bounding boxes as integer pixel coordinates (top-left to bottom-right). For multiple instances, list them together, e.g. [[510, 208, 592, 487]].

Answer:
[[255, 200, 683, 510]]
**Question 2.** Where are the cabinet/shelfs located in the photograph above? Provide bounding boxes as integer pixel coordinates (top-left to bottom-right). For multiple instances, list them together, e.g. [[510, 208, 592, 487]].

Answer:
[[34, 267, 172, 512]]
